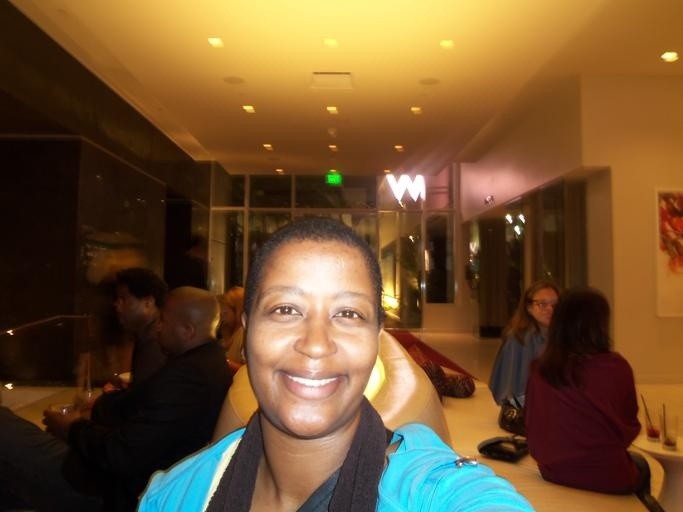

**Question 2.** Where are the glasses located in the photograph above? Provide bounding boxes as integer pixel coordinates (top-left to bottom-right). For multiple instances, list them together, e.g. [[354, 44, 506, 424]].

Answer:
[[530, 299, 556, 307]]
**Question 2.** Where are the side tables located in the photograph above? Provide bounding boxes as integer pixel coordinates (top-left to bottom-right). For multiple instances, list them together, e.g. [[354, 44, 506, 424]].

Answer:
[[632, 433, 683, 512]]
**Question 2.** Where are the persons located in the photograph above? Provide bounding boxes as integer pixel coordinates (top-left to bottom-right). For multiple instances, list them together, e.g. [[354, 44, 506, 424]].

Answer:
[[490, 281, 650, 495], [138, 214, 533, 511]]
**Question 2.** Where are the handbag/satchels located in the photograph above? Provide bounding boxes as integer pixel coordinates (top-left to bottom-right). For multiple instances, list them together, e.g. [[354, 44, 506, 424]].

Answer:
[[478, 436, 529, 463], [498, 402, 527, 436]]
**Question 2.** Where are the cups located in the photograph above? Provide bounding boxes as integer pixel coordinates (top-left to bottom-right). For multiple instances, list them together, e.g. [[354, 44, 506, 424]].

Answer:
[[48, 402, 75, 417], [661, 413, 679, 450], [643, 404, 660, 442]]
